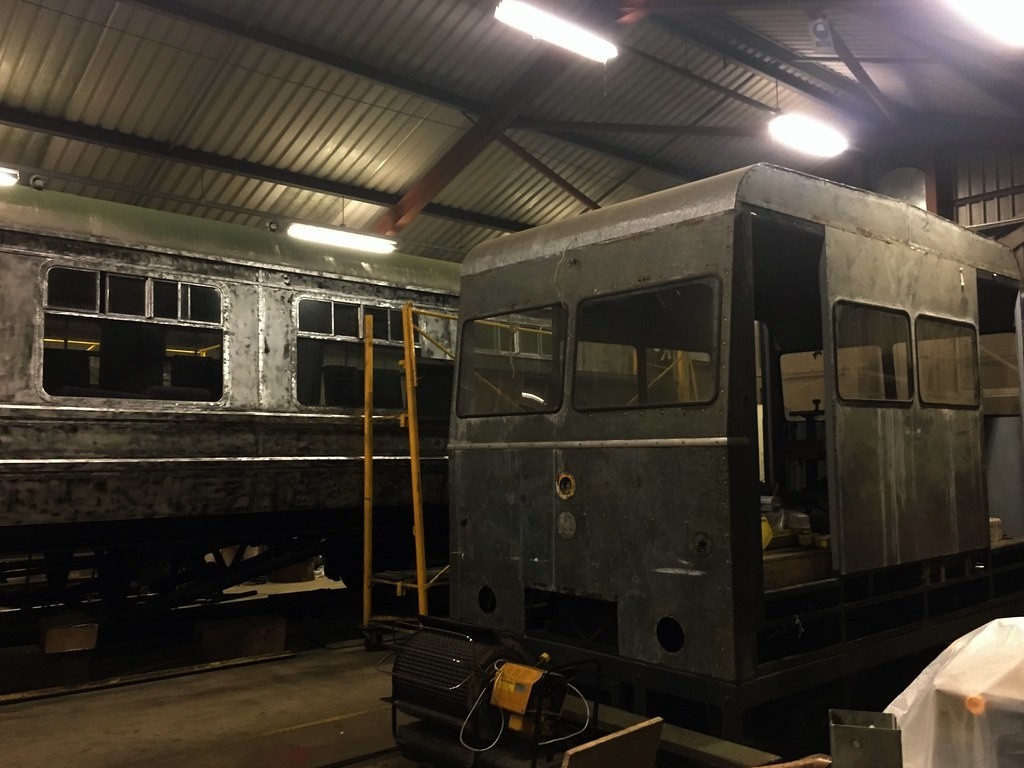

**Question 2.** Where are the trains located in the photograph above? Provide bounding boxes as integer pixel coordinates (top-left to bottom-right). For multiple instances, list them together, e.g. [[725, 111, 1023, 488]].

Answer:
[[0, 167, 562, 663]]
